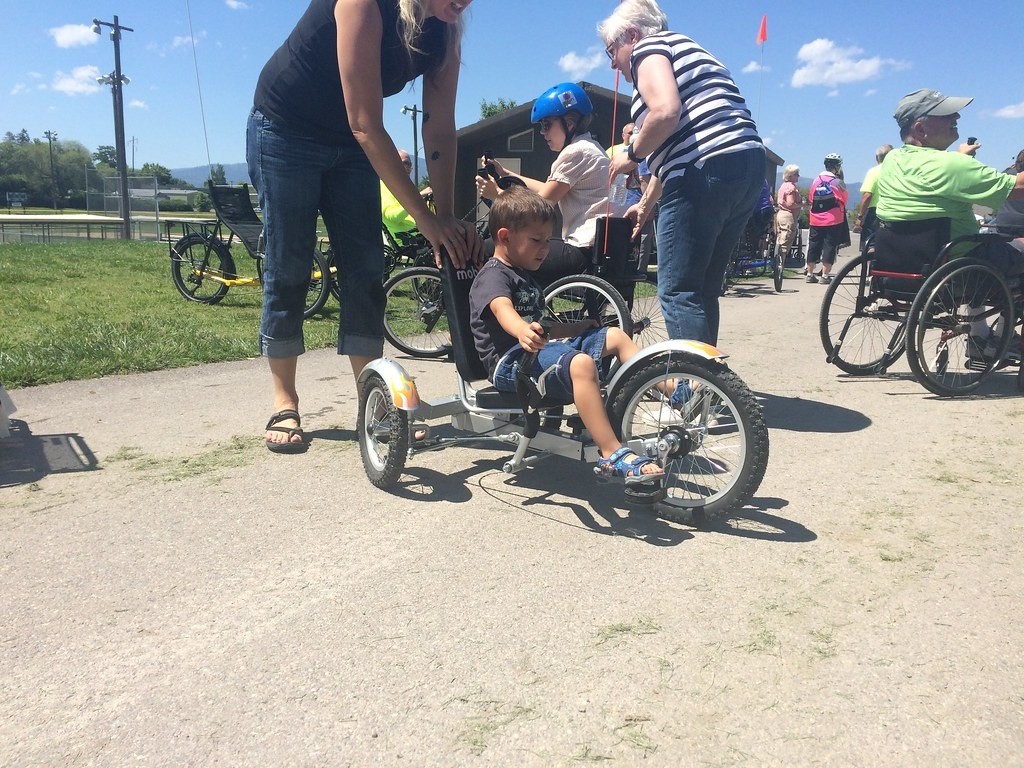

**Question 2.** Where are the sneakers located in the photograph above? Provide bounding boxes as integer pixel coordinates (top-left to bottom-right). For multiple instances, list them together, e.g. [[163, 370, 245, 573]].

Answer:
[[965, 328, 993, 360], [819, 276, 832, 284], [806, 275, 818, 283], [984, 330, 1022, 360]]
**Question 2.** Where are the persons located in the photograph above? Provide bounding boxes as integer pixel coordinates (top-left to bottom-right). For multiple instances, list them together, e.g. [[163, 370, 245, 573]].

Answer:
[[380, 150, 437, 250], [476, 83, 628, 286], [853, 144, 893, 286], [974, 150, 1024, 237], [606, 123, 658, 275], [774, 165, 808, 271], [745, 178, 774, 271], [597, 0, 766, 406], [874, 89, 1024, 361], [245, 0, 487, 450], [469, 185, 728, 487], [803, 153, 851, 285]]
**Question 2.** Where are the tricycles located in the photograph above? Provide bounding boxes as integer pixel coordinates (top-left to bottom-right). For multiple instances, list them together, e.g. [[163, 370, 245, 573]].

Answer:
[[719, 221, 784, 297], [165, 146, 652, 388], [354, 245, 770, 527]]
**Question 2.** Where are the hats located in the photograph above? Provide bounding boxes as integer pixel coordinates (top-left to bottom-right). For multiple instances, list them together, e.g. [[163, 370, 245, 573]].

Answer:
[[892, 88, 973, 128]]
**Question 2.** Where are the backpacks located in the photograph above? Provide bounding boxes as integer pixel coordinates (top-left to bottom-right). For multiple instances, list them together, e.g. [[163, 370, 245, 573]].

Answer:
[[810, 181, 839, 213]]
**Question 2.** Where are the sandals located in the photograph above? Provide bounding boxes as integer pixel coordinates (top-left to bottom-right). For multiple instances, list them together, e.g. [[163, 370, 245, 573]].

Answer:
[[594, 447, 666, 484], [668, 361, 728, 421]]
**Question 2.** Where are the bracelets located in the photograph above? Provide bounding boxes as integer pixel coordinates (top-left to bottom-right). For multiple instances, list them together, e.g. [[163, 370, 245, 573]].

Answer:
[[628, 142, 646, 163], [857, 213, 864, 220]]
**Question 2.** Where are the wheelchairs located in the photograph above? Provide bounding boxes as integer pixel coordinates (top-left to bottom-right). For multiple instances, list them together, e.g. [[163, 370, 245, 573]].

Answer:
[[817, 204, 1024, 399]]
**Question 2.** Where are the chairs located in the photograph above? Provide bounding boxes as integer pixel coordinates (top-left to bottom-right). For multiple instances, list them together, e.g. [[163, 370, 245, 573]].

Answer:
[[439, 243, 562, 413]]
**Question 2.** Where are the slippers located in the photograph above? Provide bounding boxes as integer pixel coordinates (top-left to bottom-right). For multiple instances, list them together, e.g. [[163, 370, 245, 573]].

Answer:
[[265, 409, 306, 453], [365, 419, 429, 443]]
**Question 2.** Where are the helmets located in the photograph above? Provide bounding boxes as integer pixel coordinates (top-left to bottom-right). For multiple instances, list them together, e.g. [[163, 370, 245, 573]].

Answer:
[[531, 82, 593, 124], [825, 153, 844, 164]]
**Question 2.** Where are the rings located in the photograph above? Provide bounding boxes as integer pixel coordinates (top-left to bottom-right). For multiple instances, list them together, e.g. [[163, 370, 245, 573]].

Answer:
[[451, 239, 457, 243]]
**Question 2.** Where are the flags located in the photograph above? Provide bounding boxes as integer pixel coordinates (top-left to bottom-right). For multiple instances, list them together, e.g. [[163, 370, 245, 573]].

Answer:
[[757, 15, 767, 43]]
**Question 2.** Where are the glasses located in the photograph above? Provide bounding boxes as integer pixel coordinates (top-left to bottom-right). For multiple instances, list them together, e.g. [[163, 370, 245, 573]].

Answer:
[[628, 131, 633, 135], [604, 29, 627, 61], [539, 118, 559, 130]]
